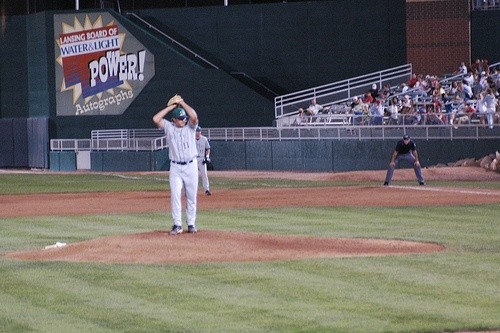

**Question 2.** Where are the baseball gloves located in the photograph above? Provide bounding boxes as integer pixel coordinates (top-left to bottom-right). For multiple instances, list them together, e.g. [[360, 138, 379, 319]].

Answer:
[[166, 95, 183, 108], [202, 159, 214, 171]]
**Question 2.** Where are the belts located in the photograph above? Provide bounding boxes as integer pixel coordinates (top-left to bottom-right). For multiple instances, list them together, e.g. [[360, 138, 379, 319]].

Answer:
[[170, 160, 193, 165]]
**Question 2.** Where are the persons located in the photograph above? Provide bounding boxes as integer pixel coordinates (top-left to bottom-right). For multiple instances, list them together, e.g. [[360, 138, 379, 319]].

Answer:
[[185, 126, 212, 196], [153, 95, 199, 235], [293, 59, 500, 129], [384, 134, 424, 186]]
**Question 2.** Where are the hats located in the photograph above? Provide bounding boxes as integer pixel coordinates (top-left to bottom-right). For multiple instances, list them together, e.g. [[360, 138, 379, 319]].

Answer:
[[403, 133, 410, 140], [371, 84, 376, 89]]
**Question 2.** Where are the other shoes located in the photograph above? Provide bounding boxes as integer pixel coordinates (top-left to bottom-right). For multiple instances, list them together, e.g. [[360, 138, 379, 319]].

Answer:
[[419, 182, 424, 185], [205, 189, 211, 195], [188, 225, 197, 233], [168, 225, 183, 235], [383, 181, 389, 185]]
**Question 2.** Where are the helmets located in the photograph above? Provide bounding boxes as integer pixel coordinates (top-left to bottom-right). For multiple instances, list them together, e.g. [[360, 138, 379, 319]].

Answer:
[[196, 126, 201, 131], [170, 108, 187, 120]]
